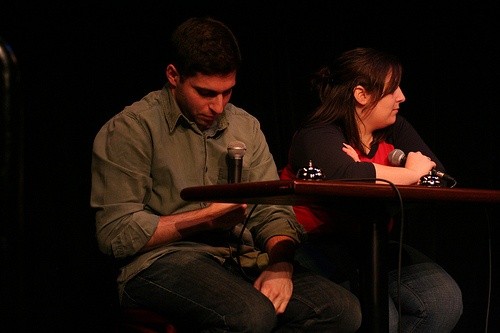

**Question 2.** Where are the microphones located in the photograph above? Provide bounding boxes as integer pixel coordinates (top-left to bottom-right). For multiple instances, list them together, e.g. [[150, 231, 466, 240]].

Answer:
[[389, 149, 455, 182], [226, 144, 246, 183]]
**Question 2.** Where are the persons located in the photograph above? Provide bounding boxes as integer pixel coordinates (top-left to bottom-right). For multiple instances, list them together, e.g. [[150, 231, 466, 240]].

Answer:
[[88, 41, 363, 331], [279, 45, 466, 330]]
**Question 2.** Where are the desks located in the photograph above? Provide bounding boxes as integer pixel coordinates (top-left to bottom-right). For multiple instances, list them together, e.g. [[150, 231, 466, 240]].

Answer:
[[181, 180, 500, 333]]
